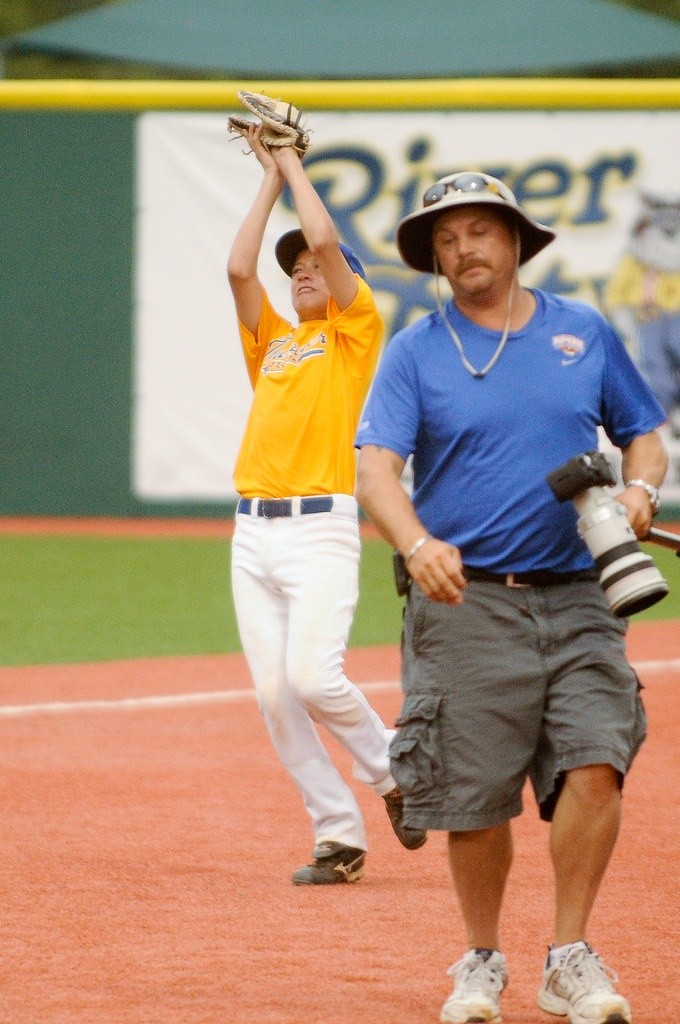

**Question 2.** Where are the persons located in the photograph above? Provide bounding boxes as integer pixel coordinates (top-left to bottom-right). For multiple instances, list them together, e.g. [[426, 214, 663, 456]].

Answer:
[[226, 122, 427, 887], [355, 170, 668, 1024]]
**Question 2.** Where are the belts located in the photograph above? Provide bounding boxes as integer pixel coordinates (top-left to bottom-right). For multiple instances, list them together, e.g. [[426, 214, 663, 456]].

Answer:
[[461, 564, 599, 588], [237, 494, 334, 519]]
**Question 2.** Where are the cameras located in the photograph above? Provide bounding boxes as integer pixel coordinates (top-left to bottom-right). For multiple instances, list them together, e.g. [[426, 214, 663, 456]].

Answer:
[[547, 449, 669, 618]]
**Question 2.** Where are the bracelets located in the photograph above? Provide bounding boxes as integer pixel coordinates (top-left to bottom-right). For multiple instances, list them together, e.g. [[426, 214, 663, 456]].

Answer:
[[403, 534, 433, 564]]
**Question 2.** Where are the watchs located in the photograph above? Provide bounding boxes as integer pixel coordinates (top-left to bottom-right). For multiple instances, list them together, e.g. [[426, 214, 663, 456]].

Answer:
[[626, 479, 663, 515]]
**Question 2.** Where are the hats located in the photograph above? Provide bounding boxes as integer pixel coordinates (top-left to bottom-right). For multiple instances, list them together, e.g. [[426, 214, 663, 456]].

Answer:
[[275, 230, 366, 283], [395, 173, 557, 273]]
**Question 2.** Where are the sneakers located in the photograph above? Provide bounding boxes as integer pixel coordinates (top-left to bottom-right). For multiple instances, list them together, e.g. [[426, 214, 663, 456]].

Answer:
[[439, 947, 508, 1024], [292, 841, 366, 886], [382, 785, 428, 850], [536, 942, 632, 1024]]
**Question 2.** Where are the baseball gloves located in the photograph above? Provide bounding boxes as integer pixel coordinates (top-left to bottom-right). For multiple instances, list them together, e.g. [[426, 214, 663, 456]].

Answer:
[[227, 89, 310, 159]]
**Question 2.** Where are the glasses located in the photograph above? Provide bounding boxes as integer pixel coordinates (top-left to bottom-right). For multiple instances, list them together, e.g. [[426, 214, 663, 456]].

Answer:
[[423, 172, 507, 209]]
